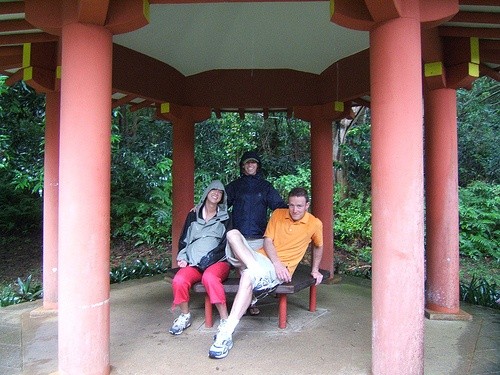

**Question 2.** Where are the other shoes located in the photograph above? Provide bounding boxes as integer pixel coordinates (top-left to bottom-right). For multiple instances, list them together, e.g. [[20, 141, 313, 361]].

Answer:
[[249, 306, 260, 315]]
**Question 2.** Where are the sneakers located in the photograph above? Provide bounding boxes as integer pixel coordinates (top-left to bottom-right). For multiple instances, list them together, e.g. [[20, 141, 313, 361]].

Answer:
[[168, 313, 191, 335], [208, 334, 233, 358], [218, 319, 227, 332], [252, 274, 279, 299]]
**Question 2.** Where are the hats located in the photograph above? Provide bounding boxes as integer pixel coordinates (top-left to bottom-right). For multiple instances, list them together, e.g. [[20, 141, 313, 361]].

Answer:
[[243, 158, 259, 164]]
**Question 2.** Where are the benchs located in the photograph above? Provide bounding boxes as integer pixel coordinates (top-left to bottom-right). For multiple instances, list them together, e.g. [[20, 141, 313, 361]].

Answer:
[[192, 267, 330, 330]]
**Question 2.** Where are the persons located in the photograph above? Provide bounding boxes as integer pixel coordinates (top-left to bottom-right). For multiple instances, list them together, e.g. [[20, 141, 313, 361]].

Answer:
[[169, 179, 235, 339], [207, 187, 324, 358], [189, 150, 288, 317]]
